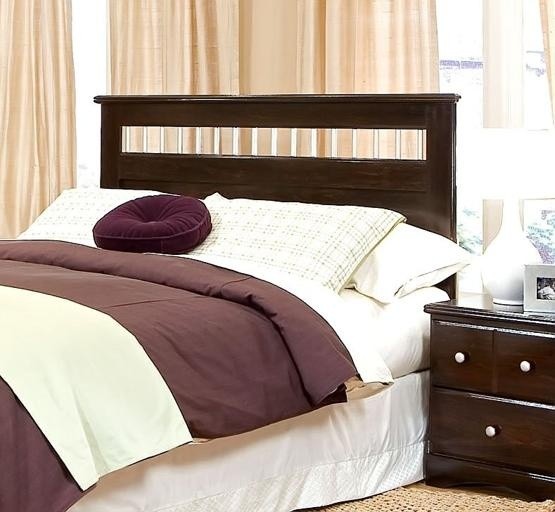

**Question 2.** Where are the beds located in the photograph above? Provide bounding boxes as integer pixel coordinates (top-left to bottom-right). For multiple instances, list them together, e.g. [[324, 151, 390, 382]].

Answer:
[[1, 93, 464, 512]]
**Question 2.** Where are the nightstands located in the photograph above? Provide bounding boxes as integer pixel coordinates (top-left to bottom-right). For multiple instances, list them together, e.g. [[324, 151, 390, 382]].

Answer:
[[421, 298, 555, 502]]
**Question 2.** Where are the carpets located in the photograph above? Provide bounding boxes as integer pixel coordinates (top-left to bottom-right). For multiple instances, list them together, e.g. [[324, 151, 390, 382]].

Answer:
[[308, 484, 554, 512]]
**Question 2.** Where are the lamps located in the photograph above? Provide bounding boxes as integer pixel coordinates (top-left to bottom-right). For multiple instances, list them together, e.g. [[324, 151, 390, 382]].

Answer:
[[468, 126, 555, 304]]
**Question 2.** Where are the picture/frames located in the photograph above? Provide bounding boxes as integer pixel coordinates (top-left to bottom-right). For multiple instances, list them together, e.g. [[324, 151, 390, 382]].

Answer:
[[522, 264, 555, 314]]
